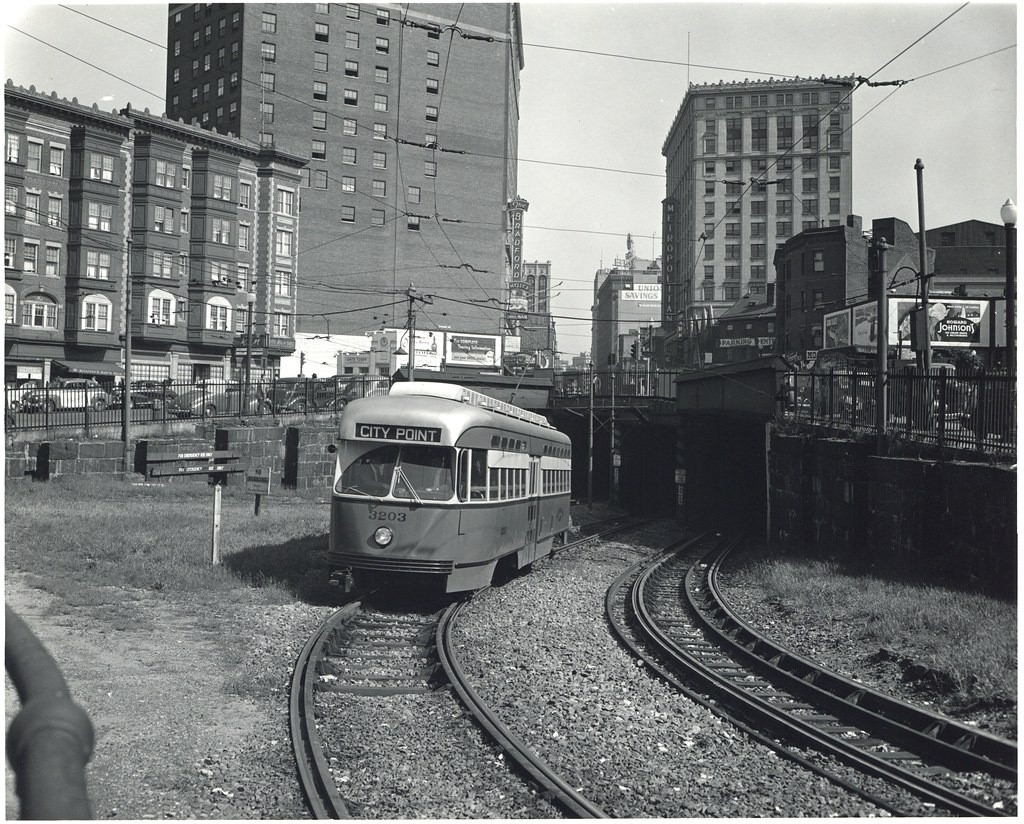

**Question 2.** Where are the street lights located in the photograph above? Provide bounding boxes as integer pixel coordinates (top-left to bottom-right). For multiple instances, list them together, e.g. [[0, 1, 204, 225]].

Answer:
[[1000, 197, 1017, 444], [124, 236, 133, 471], [393, 328, 414, 382], [244, 288, 258, 416]]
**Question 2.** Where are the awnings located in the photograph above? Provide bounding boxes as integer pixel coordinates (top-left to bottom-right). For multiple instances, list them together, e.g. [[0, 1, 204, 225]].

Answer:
[[52, 360, 132, 377]]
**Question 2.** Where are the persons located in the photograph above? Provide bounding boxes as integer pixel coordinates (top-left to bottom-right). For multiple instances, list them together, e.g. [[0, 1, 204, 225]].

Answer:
[[257, 374, 266, 418], [310, 373, 321, 413], [593, 373, 601, 396], [357, 452, 390, 494], [415, 456, 450, 491]]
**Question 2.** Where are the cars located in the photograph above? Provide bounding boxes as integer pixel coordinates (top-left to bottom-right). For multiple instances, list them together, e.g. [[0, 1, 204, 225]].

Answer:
[[167, 379, 273, 419], [563, 382, 583, 396], [264, 377, 340, 412], [903, 363, 973, 431], [817, 373, 874, 418], [782, 371, 810, 412], [111, 380, 179, 408], [23, 377, 111, 411], [336, 375, 390, 410]]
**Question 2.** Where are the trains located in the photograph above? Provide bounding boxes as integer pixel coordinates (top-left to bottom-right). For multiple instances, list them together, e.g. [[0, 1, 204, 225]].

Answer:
[[326, 380, 572, 593]]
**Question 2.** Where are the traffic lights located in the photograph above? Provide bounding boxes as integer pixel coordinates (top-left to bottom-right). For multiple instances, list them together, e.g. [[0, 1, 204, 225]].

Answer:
[[630, 340, 640, 360]]
